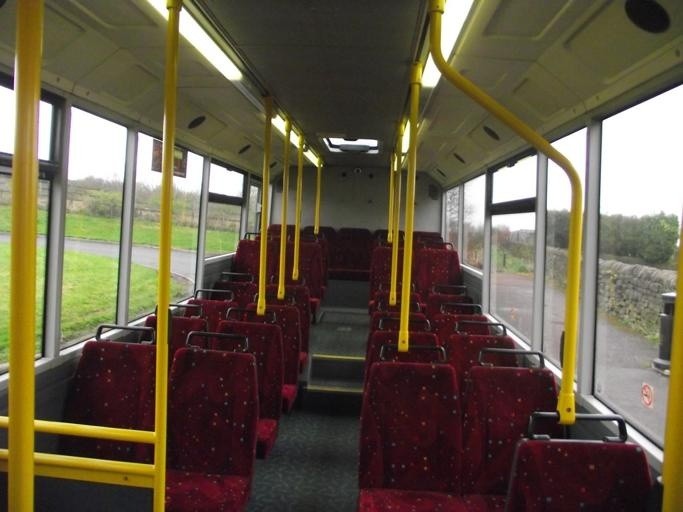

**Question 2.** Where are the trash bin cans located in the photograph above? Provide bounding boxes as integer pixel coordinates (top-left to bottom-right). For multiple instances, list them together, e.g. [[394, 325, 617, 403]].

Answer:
[[652, 292, 678, 376]]
